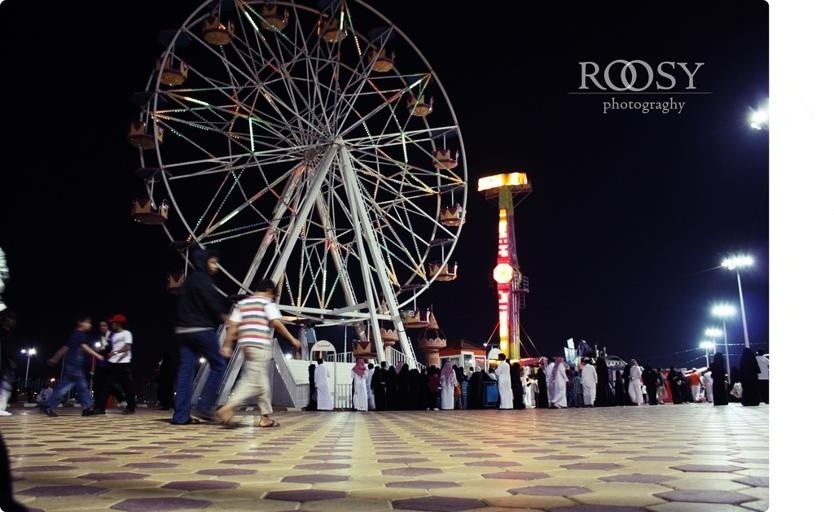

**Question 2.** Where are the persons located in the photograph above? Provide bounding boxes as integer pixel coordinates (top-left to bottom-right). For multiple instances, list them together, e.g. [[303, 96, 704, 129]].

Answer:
[[0, 249, 769, 427]]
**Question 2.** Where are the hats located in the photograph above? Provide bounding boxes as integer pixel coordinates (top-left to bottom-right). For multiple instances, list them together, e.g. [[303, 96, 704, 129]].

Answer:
[[109, 315, 126, 323]]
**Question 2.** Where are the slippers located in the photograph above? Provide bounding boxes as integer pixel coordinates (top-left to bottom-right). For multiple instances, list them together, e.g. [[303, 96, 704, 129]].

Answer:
[[259, 419, 282, 428], [215, 408, 237, 428]]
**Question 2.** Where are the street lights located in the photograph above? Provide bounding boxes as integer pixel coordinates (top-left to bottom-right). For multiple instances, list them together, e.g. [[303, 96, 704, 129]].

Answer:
[[698, 342, 713, 367], [705, 327, 723, 355], [720, 253, 755, 347], [710, 303, 737, 385], [19, 347, 36, 387], [88, 340, 101, 397]]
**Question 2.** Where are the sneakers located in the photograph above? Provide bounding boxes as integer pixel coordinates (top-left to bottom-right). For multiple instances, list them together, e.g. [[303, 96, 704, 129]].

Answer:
[[41, 403, 137, 416]]
